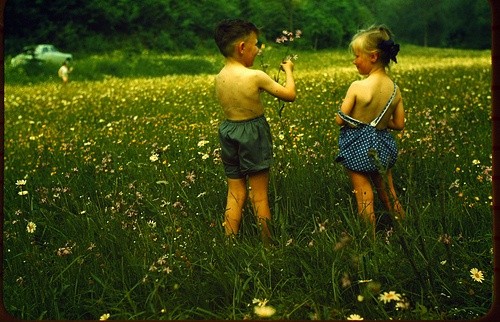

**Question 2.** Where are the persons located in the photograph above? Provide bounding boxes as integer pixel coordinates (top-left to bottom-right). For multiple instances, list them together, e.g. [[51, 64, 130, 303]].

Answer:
[[334, 25, 406, 226], [56, 61, 74, 87], [213, 19, 297, 246]]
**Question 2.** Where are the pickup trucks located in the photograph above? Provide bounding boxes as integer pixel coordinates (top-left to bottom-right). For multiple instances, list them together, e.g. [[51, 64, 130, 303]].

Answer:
[[11, 45, 73, 66]]
